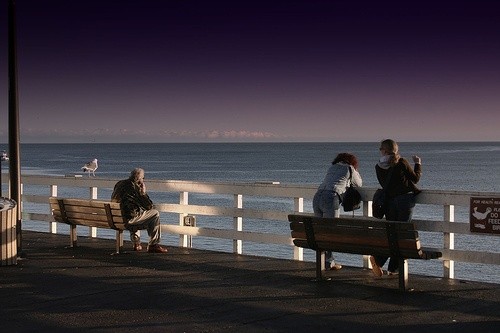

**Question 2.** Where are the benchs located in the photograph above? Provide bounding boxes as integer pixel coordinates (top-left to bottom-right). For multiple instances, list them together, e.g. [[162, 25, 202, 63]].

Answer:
[[287, 212, 443, 294], [49, 196, 130, 254]]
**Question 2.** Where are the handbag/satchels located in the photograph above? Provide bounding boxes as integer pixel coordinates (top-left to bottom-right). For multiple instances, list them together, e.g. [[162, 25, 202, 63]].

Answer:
[[372, 190, 383, 217], [342, 187, 360, 213]]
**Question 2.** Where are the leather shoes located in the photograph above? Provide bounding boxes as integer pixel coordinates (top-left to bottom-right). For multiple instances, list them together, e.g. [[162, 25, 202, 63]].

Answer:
[[132, 244, 142, 251], [149, 246, 168, 253]]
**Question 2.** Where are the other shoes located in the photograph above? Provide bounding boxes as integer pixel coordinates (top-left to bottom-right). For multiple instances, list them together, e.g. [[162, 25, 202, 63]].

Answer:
[[390, 270, 399, 279], [329, 263, 344, 271], [368, 257, 382, 278]]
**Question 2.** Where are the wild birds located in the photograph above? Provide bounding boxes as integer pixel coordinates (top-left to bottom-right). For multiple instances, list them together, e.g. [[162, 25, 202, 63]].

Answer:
[[81, 158, 98, 177]]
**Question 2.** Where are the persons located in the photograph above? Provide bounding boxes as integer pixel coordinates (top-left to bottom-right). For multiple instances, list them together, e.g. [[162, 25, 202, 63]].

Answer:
[[370, 139, 422, 278], [111, 168, 168, 253], [313, 153, 362, 270]]
